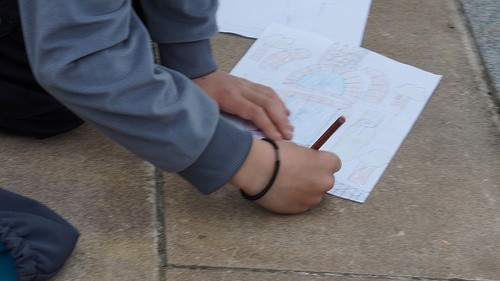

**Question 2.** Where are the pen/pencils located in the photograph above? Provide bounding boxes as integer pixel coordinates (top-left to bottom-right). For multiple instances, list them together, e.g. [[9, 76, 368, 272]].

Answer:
[[311, 117, 346, 150]]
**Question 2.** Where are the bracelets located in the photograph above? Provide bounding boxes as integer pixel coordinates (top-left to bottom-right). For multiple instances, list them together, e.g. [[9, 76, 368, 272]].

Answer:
[[240, 137, 280, 200]]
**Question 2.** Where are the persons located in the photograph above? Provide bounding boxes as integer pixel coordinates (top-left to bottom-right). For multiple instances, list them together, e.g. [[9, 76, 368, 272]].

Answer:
[[0, 0, 342, 214]]
